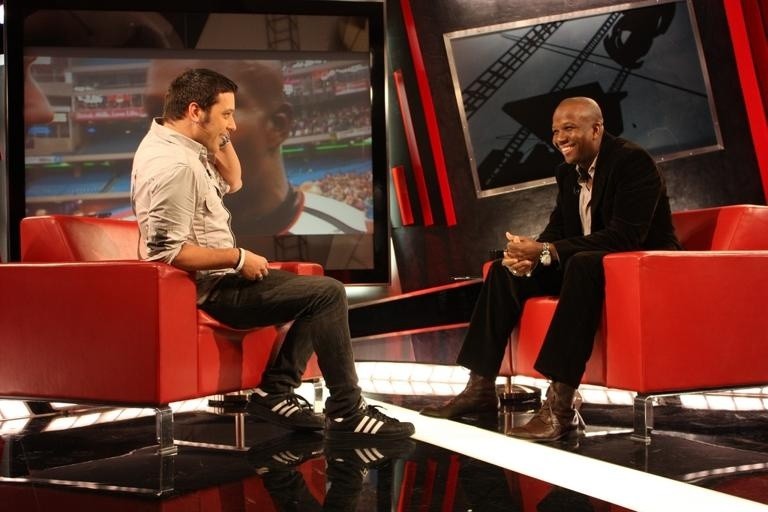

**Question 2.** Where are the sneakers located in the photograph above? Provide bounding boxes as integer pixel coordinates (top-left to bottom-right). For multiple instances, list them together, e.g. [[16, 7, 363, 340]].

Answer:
[[247, 393, 416, 439], [247, 431, 416, 468]]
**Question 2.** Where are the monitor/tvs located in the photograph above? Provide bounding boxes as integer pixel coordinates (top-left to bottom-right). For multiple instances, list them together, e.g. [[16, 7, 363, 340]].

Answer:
[[444, 0, 725, 200], [3, 0, 391, 288], [25, 46, 374, 246]]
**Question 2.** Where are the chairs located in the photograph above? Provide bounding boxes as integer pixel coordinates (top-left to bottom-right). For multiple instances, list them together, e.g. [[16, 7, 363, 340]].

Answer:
[[497, 440, 767, 511], [0, 215, 325, 460], [482, 204, 768, 445], [1, 457, 327, 511]]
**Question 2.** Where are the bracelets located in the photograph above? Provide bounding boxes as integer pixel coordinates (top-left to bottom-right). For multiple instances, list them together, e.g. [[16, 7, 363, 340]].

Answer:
[[232, 246, 245, 271]]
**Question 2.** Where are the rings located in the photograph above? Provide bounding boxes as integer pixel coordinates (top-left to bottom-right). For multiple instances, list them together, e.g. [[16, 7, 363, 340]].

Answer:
[[510, 268, 518, 275], [525, 271, 532, 278], [255, 273, 264, 279]]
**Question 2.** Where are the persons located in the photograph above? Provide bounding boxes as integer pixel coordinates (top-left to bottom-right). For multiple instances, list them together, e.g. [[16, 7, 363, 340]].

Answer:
[[126, 66, 416, 443], [415, 93, 688, 442], [24, 52, 374, 241], [240, 428, 405, 510]]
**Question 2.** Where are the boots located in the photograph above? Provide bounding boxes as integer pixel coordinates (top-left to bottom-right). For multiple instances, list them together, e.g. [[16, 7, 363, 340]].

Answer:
[[544, 431, 579, 451], [420, 371, 500, 419], [507, 388, 579, 442]]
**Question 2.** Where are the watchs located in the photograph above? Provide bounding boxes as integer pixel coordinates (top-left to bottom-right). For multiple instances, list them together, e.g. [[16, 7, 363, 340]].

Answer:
[[537, 241, 552, 267]]
